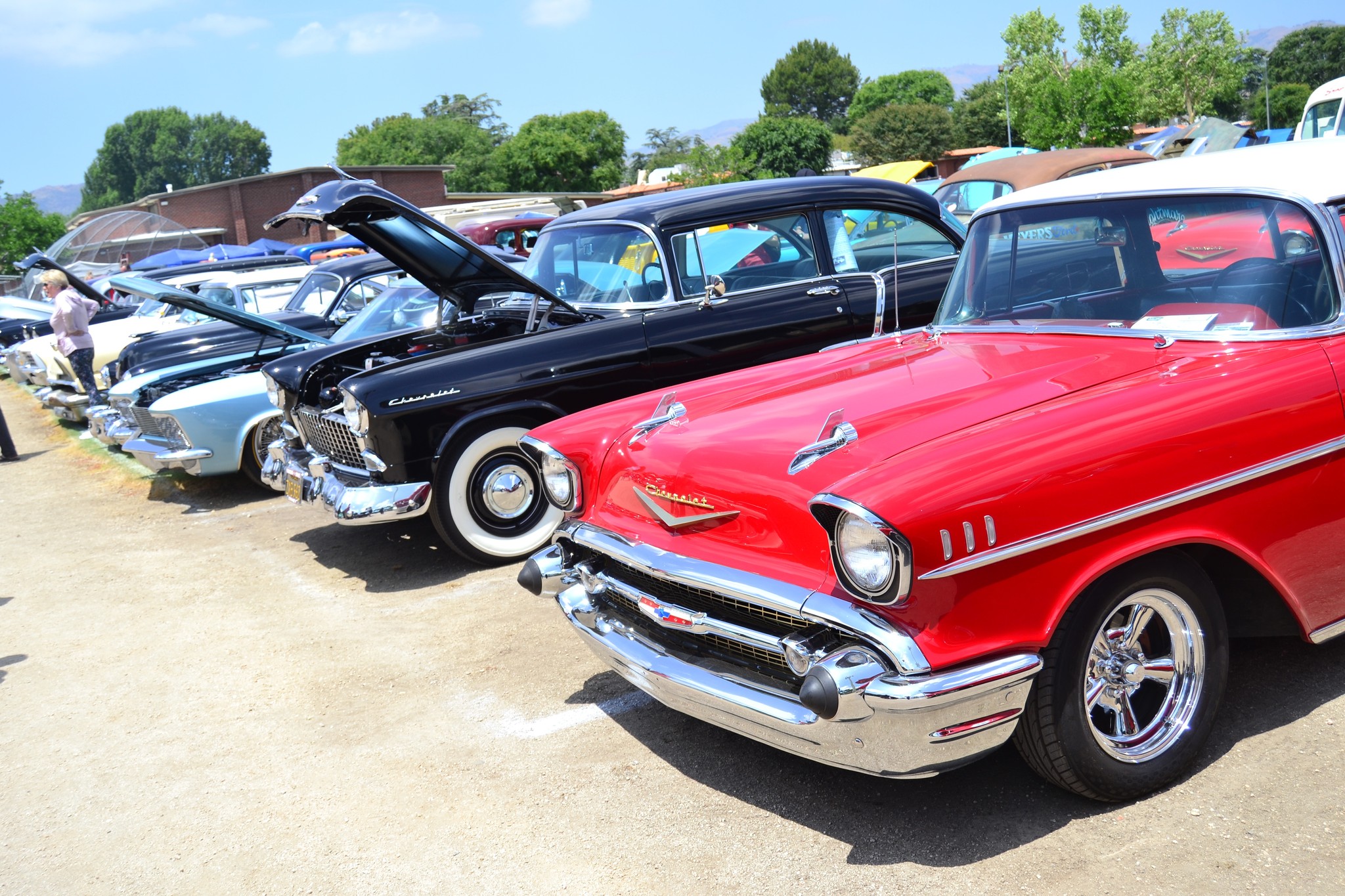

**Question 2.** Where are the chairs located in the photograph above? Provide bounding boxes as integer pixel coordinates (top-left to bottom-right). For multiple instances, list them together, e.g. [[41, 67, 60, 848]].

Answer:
[[792, 246, 928, 276]]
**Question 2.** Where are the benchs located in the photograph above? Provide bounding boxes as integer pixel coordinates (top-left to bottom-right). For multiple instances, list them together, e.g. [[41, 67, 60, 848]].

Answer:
[[1135, 284, 1330, 319], [681, 276, 806, 290]]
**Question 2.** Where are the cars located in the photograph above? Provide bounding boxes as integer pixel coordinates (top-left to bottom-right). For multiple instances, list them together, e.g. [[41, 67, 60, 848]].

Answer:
[[518, 134, 1343, 811], [107, 225, 771, 501], [779, 77, 1344, 332], [0, 210, 563, 451], [253, 173, 1126, 568]]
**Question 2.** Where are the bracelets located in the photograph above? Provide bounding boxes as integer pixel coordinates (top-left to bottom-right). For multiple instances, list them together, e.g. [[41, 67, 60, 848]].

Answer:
[[799, 233, 805, 238]]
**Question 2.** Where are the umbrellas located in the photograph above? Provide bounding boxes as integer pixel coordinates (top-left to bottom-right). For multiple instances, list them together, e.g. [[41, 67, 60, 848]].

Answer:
[[130, 238, 296, 271]]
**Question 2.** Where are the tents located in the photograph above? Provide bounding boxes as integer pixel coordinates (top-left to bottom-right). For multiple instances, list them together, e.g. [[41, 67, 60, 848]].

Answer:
[[62, 260, 120, 282]]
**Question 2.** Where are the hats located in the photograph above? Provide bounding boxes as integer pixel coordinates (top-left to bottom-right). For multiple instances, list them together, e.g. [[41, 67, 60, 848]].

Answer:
[[795, 169, 816, 177]]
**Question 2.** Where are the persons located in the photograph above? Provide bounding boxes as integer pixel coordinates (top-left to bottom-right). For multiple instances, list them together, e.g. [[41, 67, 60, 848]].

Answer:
[[39, 269, 106, 439], [85, 272, 94, 281], [785, 168, 843, 244], [119, 263, 132, 274], [0, 407, 20, 462], [728, 220, 781, 268]]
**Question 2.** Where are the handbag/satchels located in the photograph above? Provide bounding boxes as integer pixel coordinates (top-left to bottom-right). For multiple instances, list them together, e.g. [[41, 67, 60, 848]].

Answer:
[[752, 223, 780, 261]]
